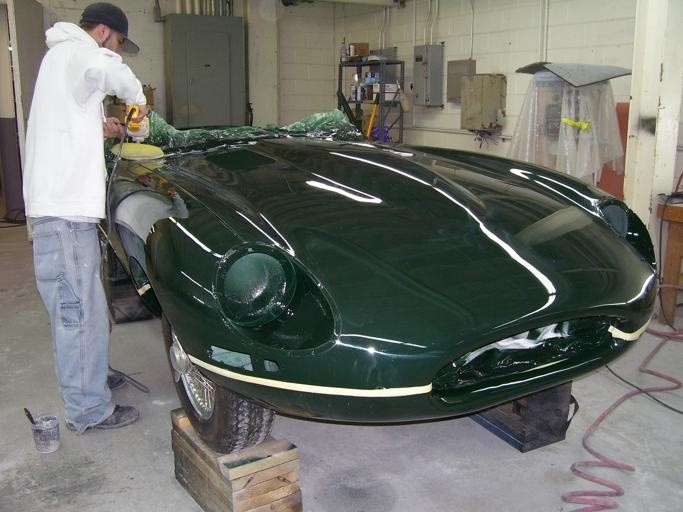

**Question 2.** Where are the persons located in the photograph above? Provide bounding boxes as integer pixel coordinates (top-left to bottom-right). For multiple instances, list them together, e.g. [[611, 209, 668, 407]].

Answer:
[[22, 3, 147, 432]]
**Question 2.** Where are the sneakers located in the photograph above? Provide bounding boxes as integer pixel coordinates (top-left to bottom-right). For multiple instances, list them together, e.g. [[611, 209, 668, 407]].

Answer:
[[64, 404, 140, 432], [106, 373, 128, 391]]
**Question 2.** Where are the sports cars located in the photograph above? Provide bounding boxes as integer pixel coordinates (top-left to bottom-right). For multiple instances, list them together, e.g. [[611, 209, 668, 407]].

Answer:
[[97, 125, 657, 456]]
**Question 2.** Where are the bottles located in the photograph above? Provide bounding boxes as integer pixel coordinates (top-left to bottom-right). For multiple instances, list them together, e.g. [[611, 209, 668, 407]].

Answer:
[[340, 40, 348, 62]]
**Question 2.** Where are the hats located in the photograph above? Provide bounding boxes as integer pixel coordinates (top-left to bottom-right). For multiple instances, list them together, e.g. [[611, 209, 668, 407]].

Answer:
[[79, 2, 140, 55]]
